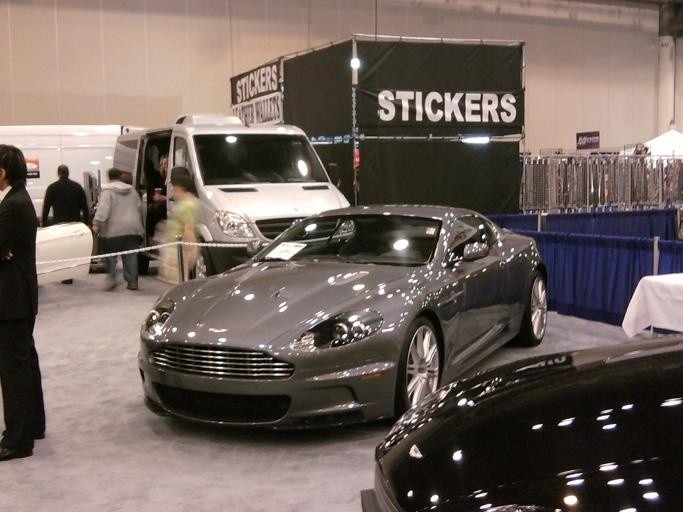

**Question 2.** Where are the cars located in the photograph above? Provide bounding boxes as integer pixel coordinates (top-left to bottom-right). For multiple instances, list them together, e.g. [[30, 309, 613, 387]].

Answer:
[[136, 205, 550, 434], [359, 333, 682, 509]]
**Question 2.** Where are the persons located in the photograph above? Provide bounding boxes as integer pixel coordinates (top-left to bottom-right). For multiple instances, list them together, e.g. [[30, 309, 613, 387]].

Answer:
[[145, 152, 168, 239], [0, 145, 48, 463], [41, 164, 89, 285], [159, 167, 203, 300], [92, 168, 146, 290]]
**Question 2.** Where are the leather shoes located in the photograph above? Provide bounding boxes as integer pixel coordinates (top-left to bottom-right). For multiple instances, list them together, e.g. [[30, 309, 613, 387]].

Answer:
[[0, 430, 44, 461]]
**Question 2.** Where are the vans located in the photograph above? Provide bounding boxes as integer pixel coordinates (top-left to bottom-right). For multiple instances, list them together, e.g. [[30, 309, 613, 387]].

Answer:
[[113, 114, 355, 283]]
